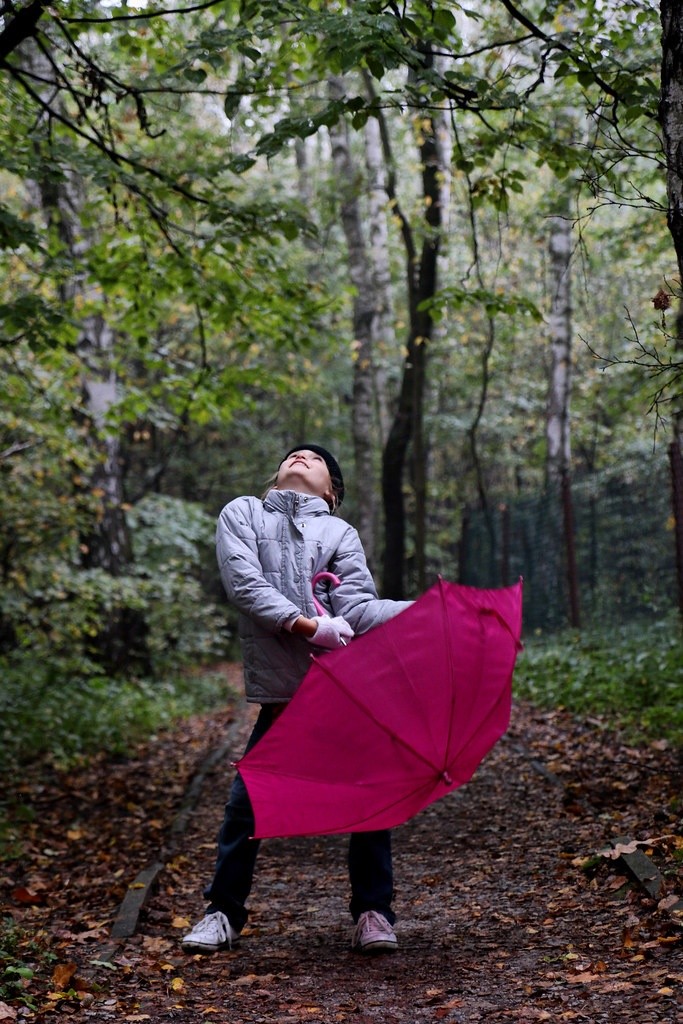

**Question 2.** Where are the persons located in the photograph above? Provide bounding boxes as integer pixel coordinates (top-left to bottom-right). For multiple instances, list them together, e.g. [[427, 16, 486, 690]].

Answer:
[[181, 444, 437, 954]]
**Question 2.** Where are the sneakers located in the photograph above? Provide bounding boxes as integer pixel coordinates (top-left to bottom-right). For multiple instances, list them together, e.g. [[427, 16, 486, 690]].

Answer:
[[351, 911, 399, 950], [182, 911, 239, 951]]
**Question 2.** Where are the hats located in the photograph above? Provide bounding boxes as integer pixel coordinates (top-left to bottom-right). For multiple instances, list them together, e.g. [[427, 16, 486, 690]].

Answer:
[[278, 444, 344, 516]]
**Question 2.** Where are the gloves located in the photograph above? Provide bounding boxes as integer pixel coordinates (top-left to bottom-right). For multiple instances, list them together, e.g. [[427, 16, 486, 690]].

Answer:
[[304, 613, 354, 650]]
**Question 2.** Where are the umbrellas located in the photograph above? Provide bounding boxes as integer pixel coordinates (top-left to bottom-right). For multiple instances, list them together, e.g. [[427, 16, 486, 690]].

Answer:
[[232, 570, 522, 839]]
